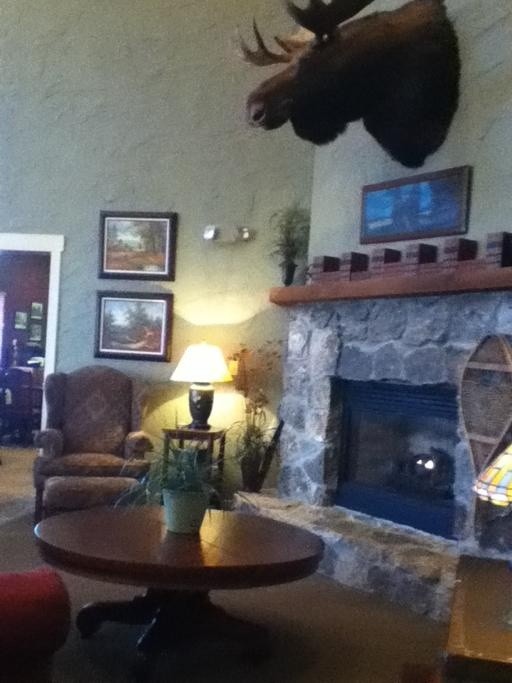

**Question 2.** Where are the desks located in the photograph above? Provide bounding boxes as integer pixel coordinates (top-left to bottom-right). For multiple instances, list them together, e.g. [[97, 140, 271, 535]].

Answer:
[[441, 553, 512, 683]]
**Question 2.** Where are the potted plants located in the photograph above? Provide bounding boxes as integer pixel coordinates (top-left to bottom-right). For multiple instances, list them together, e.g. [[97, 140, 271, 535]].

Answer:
[[268, 202, 311, 286], [232, 384, 279, 492], [110, 408, 258, 535]]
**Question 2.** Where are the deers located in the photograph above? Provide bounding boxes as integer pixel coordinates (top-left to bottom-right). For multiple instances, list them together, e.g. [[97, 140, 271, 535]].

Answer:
[[237, 0, 462, 169]]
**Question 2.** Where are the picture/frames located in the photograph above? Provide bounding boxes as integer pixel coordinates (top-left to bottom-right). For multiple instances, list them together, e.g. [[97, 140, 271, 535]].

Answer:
[[13, 300, 44, 343], [96, 209, 179, 283], [359, 164, 472, 245], [93, 291, 174, 361]]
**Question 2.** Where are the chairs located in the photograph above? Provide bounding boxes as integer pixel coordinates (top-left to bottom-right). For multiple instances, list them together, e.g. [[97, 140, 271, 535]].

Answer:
[[0, 564, 71, 683], [0, 367, 43, 448], [33, 362, 155, 524]]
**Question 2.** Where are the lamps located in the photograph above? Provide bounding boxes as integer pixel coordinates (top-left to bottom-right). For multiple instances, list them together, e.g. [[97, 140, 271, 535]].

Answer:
[[169, 342, 233, 429], [471, 442, 512, 508]]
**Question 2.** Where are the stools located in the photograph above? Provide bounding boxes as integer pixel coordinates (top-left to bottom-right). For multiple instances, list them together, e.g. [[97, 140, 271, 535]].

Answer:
[[41, 474, 146, 521], [162, 427, 227, 489]]
